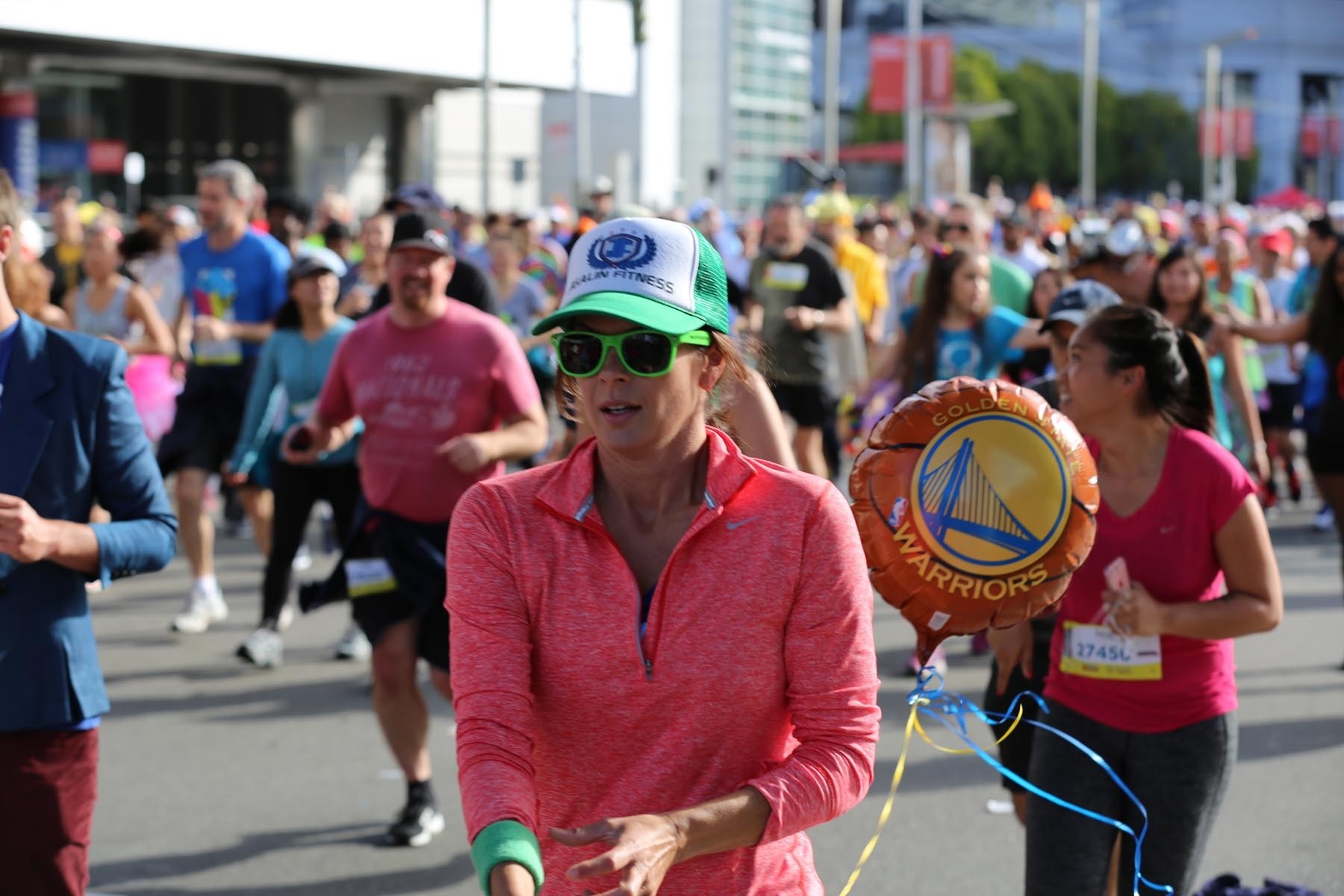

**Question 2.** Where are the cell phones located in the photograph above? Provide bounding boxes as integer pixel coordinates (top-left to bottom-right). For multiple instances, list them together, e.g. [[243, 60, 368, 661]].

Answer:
[[289, 425, 313, 452], [1102, 556, 1133, 591]]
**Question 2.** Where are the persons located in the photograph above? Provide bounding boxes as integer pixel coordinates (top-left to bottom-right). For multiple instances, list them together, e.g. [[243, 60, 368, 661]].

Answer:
[[0, 162, 1344, 896]]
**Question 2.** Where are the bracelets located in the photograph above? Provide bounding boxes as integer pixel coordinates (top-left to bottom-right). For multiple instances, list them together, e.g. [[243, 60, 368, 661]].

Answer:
[[1248, 440, 1268, 451]]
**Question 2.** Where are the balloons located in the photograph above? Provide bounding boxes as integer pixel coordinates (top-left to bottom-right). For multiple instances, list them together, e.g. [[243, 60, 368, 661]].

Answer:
[[848, 376, 1100, 669]]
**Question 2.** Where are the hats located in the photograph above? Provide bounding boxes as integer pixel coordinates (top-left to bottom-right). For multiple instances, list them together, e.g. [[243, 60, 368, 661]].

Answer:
[[531, 218, 729, 336], [1039, 280, 1126, 330], [290, 247, 347, 277], [390, 211, 454, 257]]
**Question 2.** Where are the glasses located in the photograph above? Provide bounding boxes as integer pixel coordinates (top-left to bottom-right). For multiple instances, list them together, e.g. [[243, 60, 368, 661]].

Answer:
[[549, 330, 711, 378]]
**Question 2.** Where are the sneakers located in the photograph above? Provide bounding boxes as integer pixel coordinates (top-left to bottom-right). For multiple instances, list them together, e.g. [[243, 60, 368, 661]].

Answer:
[[910, 647, 945, 682], [237, 626, 282, 672], [173, 587, 227, 632], [375, 804, 443, 847], [338, 623, 371, 657]]
